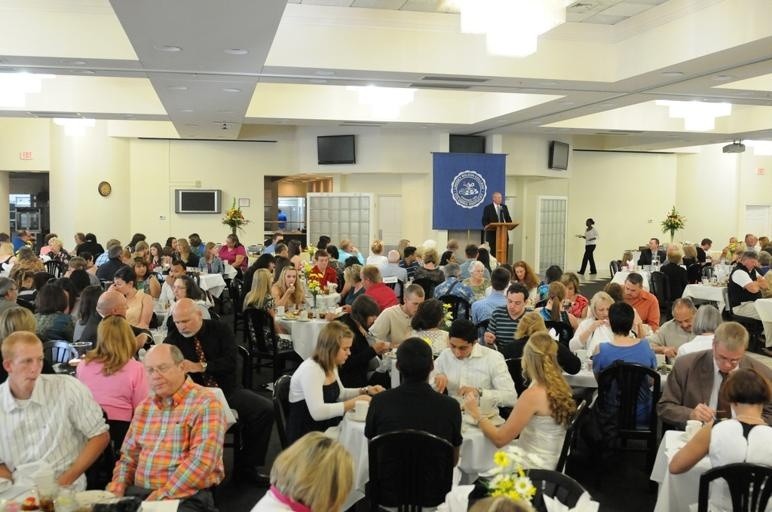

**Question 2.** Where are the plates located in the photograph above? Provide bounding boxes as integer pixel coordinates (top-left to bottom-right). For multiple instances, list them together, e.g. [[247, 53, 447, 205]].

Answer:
[[283, 317, 296, 320], [299, 319, 311, 322], [383, 352, 398, 359], [347, 414, 368, 421], [74, 490, 116, 508], [463, 416, 505, 426]]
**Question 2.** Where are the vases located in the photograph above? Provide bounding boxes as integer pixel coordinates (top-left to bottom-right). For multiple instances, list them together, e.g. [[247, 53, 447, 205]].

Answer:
[[671, 231, 675, 242], [232, 228, 238, 237]]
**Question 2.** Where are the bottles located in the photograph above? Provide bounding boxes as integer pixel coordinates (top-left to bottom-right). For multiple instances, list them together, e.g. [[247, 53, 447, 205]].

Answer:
[[662, 362, 666, 375]]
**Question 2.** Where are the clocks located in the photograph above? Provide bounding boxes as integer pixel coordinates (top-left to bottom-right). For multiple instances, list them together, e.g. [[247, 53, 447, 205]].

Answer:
[[98, 181, 111, 196]]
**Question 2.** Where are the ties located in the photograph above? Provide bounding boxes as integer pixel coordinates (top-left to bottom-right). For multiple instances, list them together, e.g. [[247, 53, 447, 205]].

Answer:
[[497, 206, 500, 220], [652, 252, 654, 262], [716, 370, 731, 420], [194, 334, 220, 389]]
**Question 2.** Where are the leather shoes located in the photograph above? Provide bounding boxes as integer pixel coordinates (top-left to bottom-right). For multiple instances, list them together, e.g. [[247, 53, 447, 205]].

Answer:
[[237, 464, 271, 489]]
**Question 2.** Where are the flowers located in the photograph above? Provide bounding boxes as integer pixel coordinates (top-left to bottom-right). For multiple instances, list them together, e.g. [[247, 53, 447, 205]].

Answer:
[[223, 199, 248, 237], [662, 208, 684, 240]]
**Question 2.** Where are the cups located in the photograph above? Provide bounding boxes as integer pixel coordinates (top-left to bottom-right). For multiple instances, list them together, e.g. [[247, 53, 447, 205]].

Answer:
[[318, 304, 327, 319], [328, 307, 336, 314], [711, 278, 717, 286], [655, 354, 666, 367], [355, 400, 369, 418], [157, 326, 168, 336], [37, 486, 55, 512], [301, 311, 308, 320], [636, 266, 642, 271], [622, 266, 628, 272], [277, 305, 284, 314], [479, 396, 491, 415], [585, 360, 593, 373], [203, 267, 208, 275], [153, 335, 163, 345], [324, 285, 329, 296], [685, 420, 703, 440], [701, 276, 707, 284], [31, 469, 55, 496], [60, 484, 77, 505], [575, 349, 589, 368]]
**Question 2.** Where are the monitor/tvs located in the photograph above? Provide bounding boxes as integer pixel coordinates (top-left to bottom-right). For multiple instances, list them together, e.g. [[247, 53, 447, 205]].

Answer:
[[548, 140, 569, 170], [317, 134, 356, 164], [175, 189, 221, 214], [449, 134, 485, 153]]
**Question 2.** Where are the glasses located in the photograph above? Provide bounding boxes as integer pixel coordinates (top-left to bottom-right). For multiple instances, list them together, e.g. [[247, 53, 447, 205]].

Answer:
[[172, 286, 186, 291], [714, 345, 740, 364], [147, 360, 183, 375]]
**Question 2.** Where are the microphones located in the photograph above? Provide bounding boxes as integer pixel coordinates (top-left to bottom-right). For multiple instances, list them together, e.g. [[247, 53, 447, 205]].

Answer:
[[498, 207, 505, 223]]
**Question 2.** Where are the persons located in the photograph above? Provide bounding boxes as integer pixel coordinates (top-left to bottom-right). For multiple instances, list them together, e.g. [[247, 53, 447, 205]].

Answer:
[[0, 190, 772, 512]]
